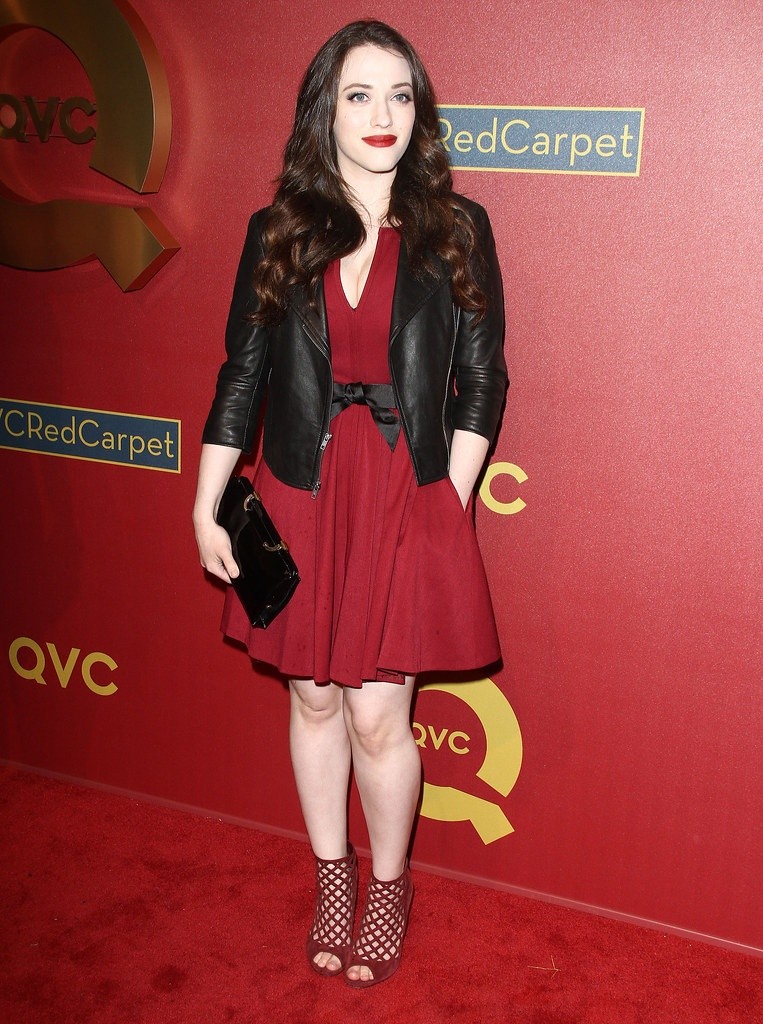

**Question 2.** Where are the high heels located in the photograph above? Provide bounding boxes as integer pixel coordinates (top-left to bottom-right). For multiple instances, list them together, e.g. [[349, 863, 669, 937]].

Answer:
[[306, 843, 360, 975], [344, 858, 414, 988]]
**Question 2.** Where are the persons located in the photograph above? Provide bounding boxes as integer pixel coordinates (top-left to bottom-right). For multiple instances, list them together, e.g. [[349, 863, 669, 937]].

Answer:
[[192, 19, 508, 989]]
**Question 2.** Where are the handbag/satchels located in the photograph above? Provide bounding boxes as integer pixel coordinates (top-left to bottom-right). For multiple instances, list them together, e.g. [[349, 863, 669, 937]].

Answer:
[[211, 477, 302, 630]]
